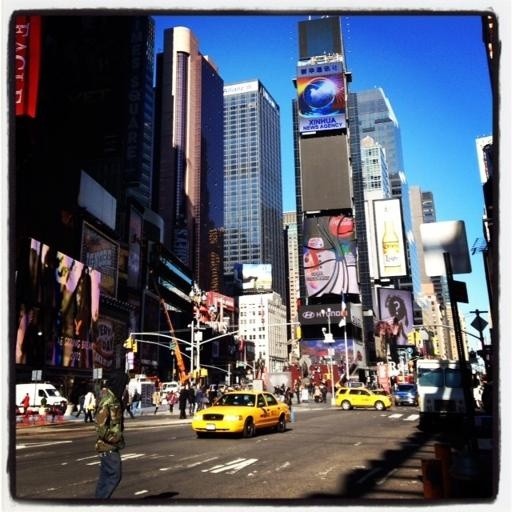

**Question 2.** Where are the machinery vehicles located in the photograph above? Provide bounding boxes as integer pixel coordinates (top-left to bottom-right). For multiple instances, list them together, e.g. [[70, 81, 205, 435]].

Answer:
[[160, 297, 188, 392]]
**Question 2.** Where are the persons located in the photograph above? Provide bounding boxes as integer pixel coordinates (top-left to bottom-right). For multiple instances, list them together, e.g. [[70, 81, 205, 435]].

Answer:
[[21, 390, 98, 423], [95, 372, 130, 499], [273, 379, 328, 411], [122, 383, 250, 419]]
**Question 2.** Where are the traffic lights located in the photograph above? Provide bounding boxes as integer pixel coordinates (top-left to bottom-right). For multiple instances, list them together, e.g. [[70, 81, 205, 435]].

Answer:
[[133, 342, 137, 352], [407, 330, 415, 345], [126, 337, 133, 348]]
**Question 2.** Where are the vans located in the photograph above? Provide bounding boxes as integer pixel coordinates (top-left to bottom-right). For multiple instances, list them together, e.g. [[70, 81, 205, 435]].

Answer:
[[15, 383, 69, 416], [393, 382, 420, 406], [332, 386, 393, 411]]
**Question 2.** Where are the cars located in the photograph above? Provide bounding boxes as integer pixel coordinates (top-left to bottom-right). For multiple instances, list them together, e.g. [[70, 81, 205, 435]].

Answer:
[[190, 390, 291, 438]]
